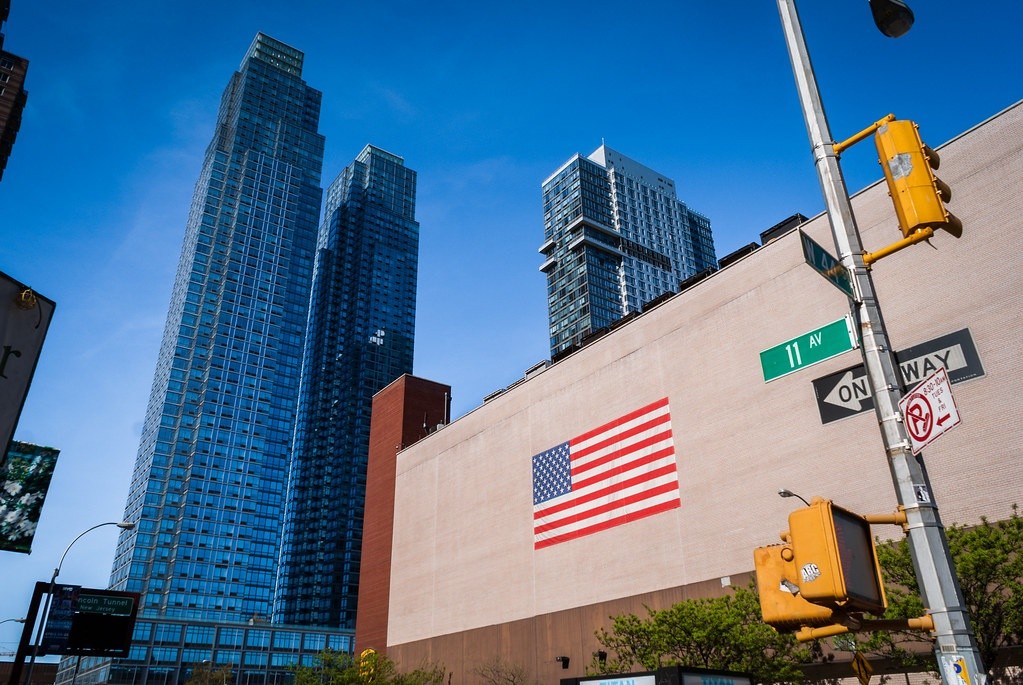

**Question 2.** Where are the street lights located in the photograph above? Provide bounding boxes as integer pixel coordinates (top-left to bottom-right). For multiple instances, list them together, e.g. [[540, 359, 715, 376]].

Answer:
[[774, 1, 984, 684], [26, 522, 134, 684]]
